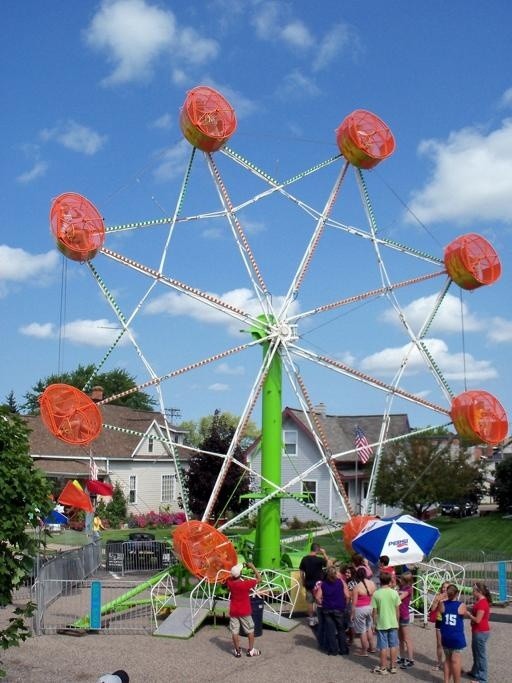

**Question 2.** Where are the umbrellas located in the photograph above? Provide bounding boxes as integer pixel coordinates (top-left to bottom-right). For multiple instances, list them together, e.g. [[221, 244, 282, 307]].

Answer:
[[351, 514, 441, 565]]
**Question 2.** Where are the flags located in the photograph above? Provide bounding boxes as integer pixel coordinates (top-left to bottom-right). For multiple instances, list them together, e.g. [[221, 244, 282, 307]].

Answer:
[[353, 426, 373, 464], [58, 481, 93, 512], [85, 480, 113, 496]]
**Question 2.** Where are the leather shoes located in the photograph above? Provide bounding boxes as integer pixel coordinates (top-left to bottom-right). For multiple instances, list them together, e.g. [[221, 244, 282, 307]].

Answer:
[[327, 651, 337, 655], [339, 650, 349, 654]]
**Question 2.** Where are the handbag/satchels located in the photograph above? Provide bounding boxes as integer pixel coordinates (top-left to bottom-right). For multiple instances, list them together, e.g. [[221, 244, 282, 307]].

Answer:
[[429, 600, 440, 621]]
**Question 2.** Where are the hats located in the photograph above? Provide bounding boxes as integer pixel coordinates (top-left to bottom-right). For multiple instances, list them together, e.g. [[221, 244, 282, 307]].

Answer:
[[96, 670, 130, 682], [231, 563, 243, 577]]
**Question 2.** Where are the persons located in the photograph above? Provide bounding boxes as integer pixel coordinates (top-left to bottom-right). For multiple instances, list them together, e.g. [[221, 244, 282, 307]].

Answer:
[[316, 566, 350, 655], [377, 556, 397, 588], [349, 568, 375, 656], [466, 583, 491, 682], [342, 566, 358, 593], [437, 585, 466, 683], [370, 571, 402, 675], [395, 572, 416, 669], [298, 543, 328, 627], [93, 514, 107, 541], [226, 561, 262, 658], [431, 582, 452, 672], [352, 550, 374, 579]]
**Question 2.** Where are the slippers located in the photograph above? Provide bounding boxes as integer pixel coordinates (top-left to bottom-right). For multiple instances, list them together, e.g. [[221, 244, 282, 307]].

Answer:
[[355, 653, 368, 656], [472, 680, 479, 682], [367, 648, 376, 652], [466, 670, 473, 676], [431, 664, 442, 669]]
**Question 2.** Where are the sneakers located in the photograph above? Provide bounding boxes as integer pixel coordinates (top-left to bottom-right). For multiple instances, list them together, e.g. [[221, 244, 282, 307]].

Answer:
[[370, 664, 387, 674], [387, 665, 397, 672], [309, 616, 319, 626], [396, 655, 404, 664], [400, 657, 415, 668], [246, 648, 261, 656], [232, 647, 242, 657]]
